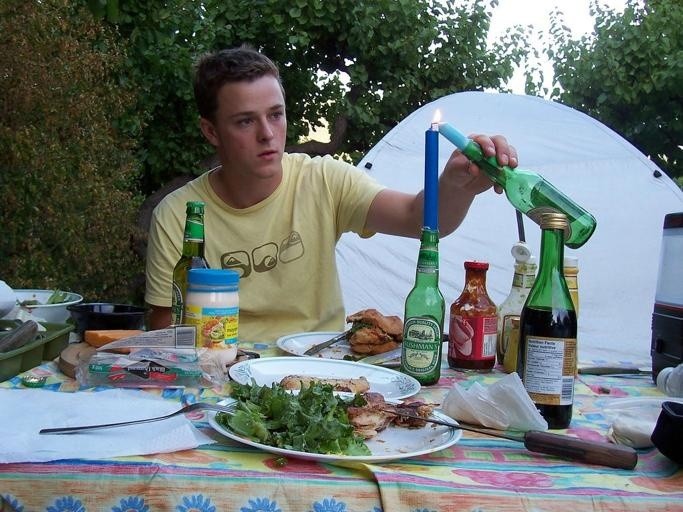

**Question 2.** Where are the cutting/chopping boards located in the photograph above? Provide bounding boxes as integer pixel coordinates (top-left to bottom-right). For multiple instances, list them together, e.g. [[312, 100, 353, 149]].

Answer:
[[59, 341, 249, 386]]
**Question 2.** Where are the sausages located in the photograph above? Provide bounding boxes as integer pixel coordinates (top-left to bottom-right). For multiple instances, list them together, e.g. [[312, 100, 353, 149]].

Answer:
[[282, 374, 369, 391]]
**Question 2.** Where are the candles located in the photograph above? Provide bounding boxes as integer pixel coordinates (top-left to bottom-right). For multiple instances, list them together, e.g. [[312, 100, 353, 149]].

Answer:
[[438, 122, 469, 151], [424, 129, 439, 231]]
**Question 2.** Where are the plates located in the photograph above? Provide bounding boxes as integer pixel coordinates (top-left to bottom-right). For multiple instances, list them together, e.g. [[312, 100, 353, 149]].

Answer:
[[228, 355, 421, 402], [276, 331, 403, 366], [209, 390, 464, 463]]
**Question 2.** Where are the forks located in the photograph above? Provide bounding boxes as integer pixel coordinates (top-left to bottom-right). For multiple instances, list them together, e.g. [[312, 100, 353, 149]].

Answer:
[[39, 403, 237, 435]]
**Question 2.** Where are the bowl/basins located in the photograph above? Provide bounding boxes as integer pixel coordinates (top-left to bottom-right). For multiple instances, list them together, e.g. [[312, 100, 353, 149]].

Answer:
[[3, 289, 83, 322], [67, 302, 152, 338]]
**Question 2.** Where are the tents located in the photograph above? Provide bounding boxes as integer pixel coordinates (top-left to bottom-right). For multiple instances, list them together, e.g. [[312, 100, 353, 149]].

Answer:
[[336, 91, 681, 372]]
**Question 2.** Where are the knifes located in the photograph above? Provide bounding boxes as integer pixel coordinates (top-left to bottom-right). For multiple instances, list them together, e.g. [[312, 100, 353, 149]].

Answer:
[[303, 323, 366, 355], [380, 408, 638, 469]]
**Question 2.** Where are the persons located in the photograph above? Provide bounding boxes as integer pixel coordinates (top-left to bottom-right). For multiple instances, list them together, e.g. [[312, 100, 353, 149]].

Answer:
[[143, 47, 519, 342]]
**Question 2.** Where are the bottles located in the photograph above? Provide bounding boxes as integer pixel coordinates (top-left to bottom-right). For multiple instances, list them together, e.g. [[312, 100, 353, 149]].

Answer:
[[184, 268, 239, 366], [499, 256, 537, 371], [403, 229, 450, 386], [562, 257, 582, 315], [450, 259, 499, 373], [517, 213, 578, 429], [460, 141, 597, 253], [171, 202, 211, 328]]
